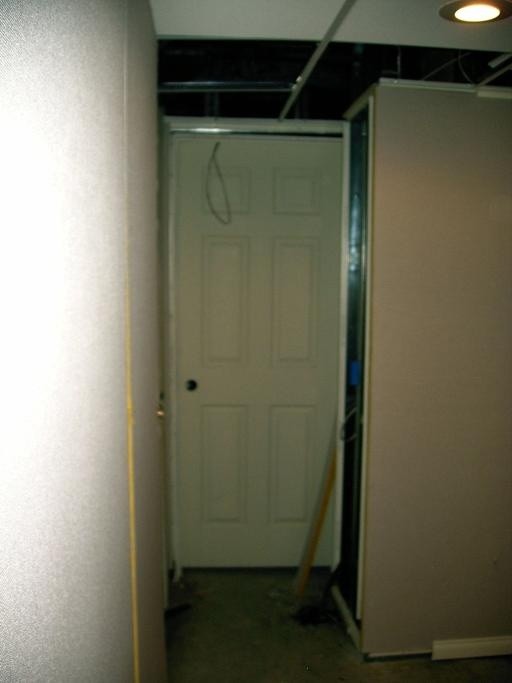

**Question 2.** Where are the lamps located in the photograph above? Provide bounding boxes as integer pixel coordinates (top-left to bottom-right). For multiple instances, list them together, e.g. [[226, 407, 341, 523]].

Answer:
[[439, 0, 508, 25]]
